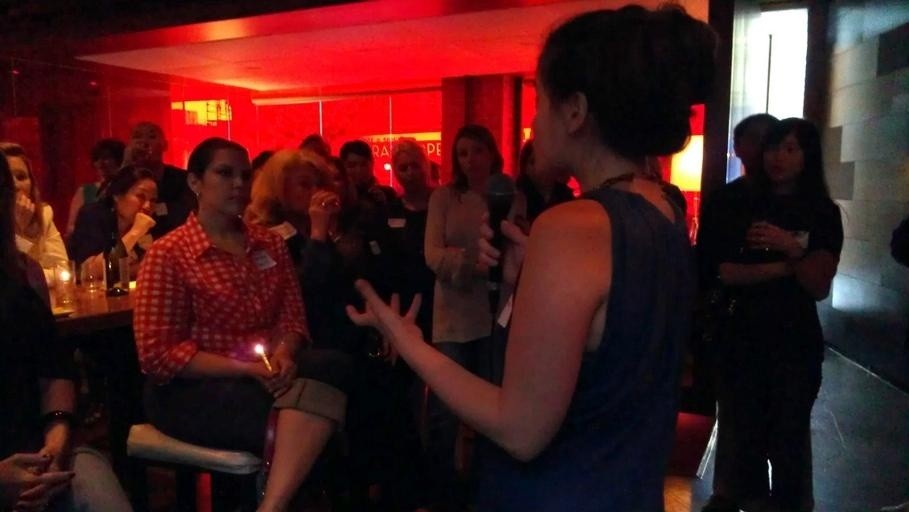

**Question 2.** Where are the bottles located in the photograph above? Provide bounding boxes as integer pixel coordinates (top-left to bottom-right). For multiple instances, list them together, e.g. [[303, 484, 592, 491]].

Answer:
[[104, 206, 130, 296]]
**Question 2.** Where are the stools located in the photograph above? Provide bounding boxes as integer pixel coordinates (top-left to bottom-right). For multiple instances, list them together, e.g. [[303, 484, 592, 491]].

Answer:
[[123, 414, 266, 512]]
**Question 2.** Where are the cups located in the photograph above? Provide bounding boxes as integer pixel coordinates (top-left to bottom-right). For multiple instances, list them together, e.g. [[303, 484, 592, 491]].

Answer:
[[52, 259, 78, 308], [81, 264, 103, 294]]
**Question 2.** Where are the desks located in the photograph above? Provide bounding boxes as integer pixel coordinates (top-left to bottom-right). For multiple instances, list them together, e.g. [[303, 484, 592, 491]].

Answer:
[[48, 284, 142, 490]]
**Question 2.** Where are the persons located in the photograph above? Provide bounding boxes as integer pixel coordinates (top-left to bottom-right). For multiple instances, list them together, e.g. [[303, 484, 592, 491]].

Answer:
[[2, 5, 843, 511]]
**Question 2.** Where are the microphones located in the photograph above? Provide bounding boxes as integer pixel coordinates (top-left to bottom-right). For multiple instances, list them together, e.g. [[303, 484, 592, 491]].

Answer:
[[483, 173, 515, 316]]
